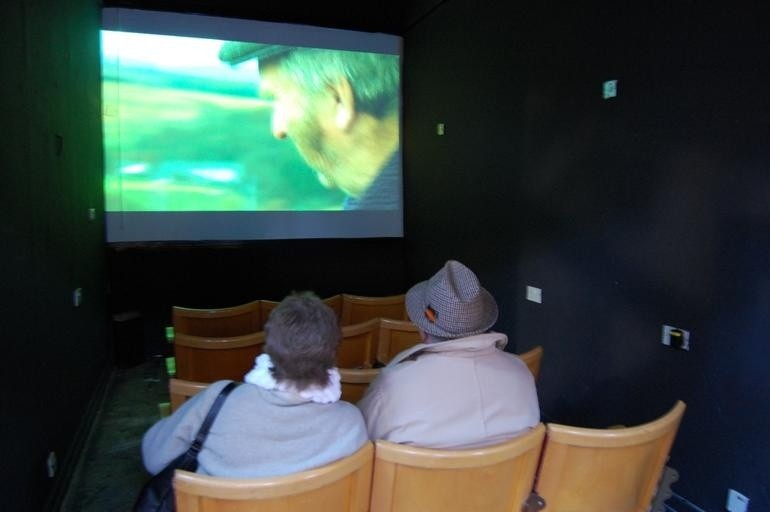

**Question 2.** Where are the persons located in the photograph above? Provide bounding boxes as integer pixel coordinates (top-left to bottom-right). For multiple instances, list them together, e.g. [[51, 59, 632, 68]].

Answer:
[[219, 41, 400, 210], [357, 260, 540, 450], [141, 290, 368, 479]]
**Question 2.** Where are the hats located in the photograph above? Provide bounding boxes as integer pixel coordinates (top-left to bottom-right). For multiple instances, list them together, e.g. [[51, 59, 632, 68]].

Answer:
[[404, 259, 499, 339], [216, 39, 296, 67]]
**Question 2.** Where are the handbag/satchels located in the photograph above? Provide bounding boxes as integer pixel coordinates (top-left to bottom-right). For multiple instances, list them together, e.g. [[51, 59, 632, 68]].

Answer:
[[131, 378, 244, 512]]
